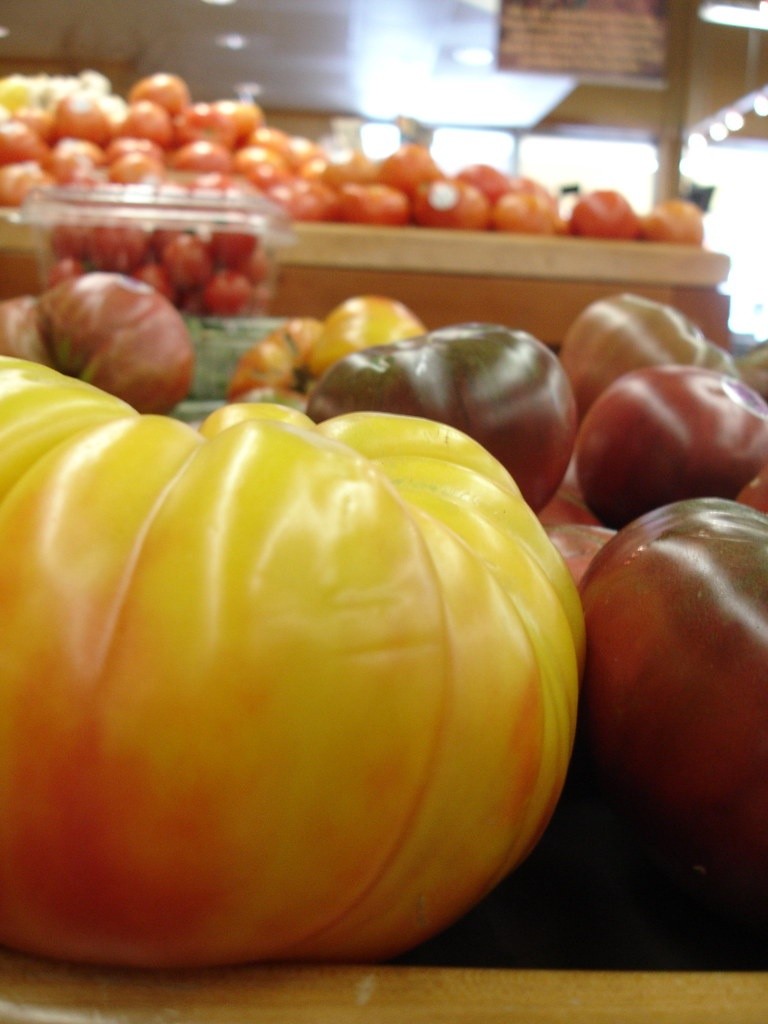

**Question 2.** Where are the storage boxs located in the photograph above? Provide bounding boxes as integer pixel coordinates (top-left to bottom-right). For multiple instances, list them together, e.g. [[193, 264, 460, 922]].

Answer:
[[11, 173, 306, 316]]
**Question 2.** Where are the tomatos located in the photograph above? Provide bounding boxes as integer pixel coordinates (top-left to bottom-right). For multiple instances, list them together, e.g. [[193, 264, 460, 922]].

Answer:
[[1, 221, 768, 973], [0, 69, 704, 249]]
